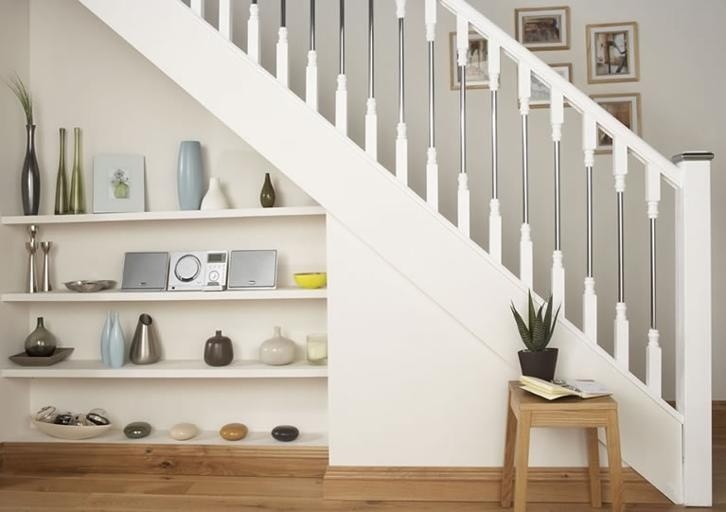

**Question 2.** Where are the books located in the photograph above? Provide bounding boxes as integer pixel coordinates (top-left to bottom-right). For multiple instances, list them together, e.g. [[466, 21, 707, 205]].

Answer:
[[519, 375, 612, 401]]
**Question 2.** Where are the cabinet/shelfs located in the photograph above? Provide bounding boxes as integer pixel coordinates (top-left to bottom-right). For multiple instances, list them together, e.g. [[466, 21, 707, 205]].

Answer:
[[0, 206, 329, 449]]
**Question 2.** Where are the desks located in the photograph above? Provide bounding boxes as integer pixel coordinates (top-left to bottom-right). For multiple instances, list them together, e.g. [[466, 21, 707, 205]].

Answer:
[[501, 381, 625, 512]]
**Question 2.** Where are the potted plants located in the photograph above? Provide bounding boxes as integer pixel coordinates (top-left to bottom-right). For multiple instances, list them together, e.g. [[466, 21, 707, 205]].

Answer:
[[510, 289, 562, 382]]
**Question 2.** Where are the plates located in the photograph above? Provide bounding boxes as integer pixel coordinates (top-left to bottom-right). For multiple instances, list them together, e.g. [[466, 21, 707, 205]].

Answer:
[[30, 417, 116, 440], [7, 347, 75, 367]]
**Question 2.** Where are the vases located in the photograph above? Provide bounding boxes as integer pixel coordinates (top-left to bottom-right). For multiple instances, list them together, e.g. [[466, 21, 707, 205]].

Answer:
[[4, 69, 40, 215], [200, 177, 231, 210], [204, 331, 233, 366], [69, 128, 85, 214], [24, 317, 56, 357], [100, 311, 125, 369], [260, 173, 275, 208], [177, 141, 203, 210], [55, 128, 69, 214], [258, 328, 298, 366]]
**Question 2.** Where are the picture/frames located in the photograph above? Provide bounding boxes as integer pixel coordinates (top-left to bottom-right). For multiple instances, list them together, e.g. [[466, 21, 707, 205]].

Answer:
[[449, 6, 644, 154]]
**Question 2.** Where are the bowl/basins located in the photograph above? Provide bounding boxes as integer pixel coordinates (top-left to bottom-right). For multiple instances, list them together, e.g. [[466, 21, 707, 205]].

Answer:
[[294, 271, 327, 290], [64, 278, 115, 293]]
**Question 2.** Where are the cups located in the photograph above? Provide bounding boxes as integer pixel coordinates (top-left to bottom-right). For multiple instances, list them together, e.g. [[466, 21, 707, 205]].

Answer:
[[305, 336, 330, 366]]
